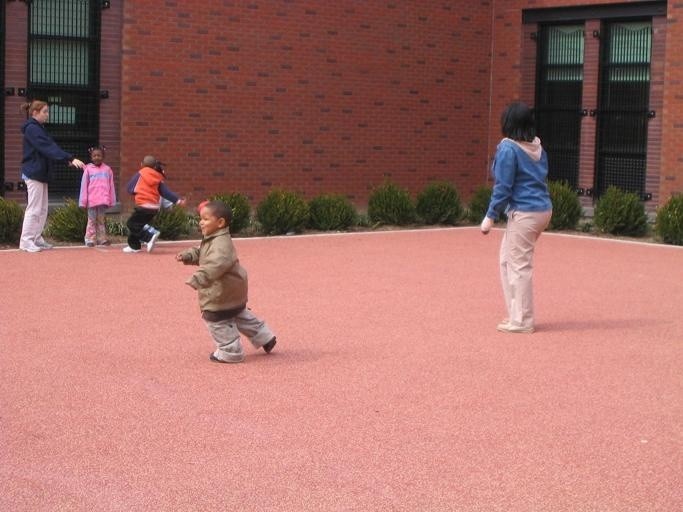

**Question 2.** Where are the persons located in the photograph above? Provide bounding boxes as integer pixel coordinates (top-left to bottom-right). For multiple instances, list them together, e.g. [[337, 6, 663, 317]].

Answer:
[[479, 100, 554, 333], [78, 145, 115, 247], [121, 154, 186, 253], [17, 100, 86, 253], [173, 200, 278, 364]]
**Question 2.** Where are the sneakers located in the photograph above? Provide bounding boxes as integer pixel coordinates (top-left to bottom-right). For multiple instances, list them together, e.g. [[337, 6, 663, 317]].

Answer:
[[146, 230, 161, 252], [262, 335, 276, 353], [209, 351, 244, 363], [97, 239, 111, 245], [86, 240, 95, 247], [122, 245, 140, 253], [20, 242, 52, 252], [496, 317, 535, 334]]
